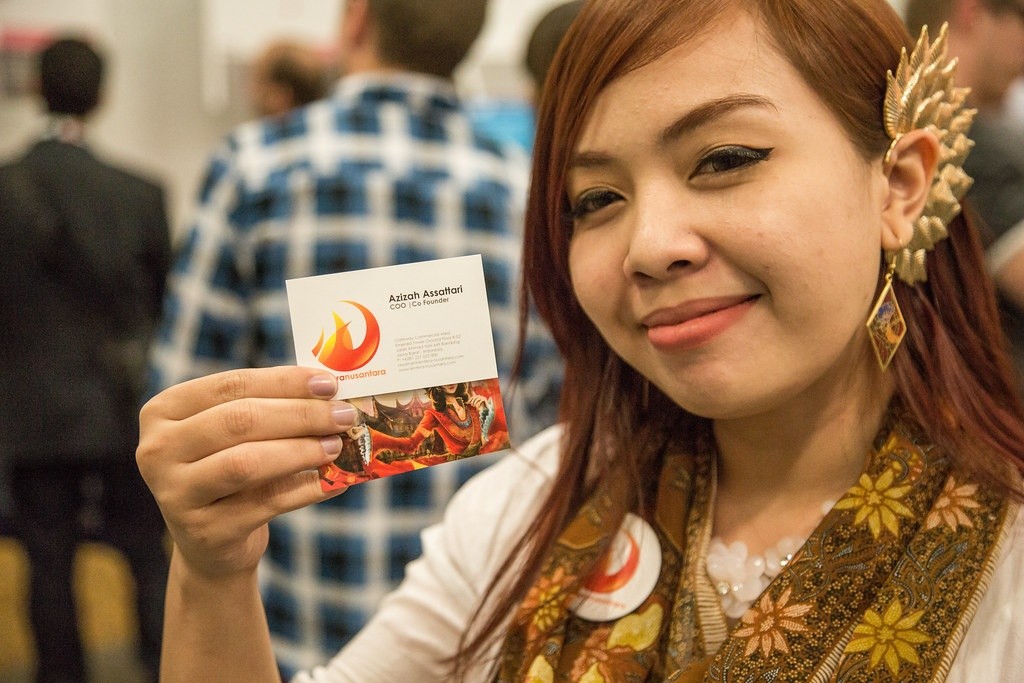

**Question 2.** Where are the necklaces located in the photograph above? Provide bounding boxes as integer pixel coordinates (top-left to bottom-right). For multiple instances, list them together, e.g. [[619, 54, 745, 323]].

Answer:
[[704, 491, 846, 621]]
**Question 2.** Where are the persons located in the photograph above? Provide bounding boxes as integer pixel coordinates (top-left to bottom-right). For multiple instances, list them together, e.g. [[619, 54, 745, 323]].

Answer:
[[903, 0, 1024, 334], [243, 44, 324, 118], [133, 1, 1023, 683], [143, 1, 568, 683], [0, 30, 177, 683]]
